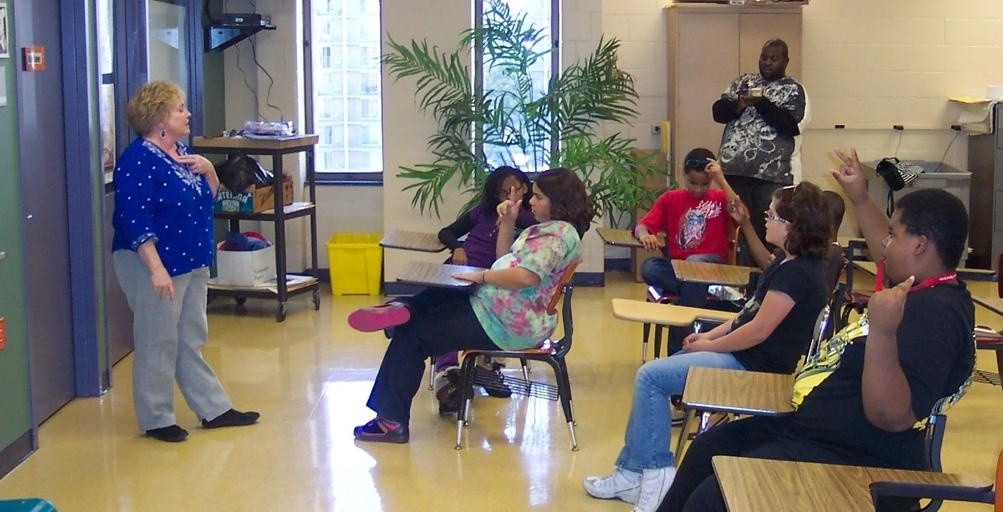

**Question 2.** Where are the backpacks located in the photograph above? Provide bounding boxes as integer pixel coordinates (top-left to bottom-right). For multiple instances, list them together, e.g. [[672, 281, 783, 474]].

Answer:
[[874, 156, 926, 191]]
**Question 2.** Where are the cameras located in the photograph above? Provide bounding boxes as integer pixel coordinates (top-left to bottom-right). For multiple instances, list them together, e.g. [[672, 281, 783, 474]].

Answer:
[[740, 83, 765, 99]]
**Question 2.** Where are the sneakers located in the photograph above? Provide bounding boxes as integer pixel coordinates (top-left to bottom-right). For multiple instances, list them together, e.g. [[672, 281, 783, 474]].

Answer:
[[688, 411, 730, 440], [668, 406, 688, 426], [201, 408, 261, 427], [580, 464, 644, 503], [146, 423, 189, 443], [472, 362, 513, 398], [434, 366, 475, 417], [632, 465, 677, 512], [353, 412, 410, 444], [347, 300, 412, 333]]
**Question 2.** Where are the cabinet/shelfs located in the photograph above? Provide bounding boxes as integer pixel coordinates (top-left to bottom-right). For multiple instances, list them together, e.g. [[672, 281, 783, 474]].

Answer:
[[191, 134, 321, 322], [664, 1, 803, 188]]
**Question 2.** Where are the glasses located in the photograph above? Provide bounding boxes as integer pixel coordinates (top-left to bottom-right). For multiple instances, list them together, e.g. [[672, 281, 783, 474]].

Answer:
[[684, 158, 712, 168], [496, 185, 523, 196]]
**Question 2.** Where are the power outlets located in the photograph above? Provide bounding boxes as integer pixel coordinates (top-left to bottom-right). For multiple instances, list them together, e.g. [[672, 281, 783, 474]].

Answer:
[[651, 124, 661, 135]]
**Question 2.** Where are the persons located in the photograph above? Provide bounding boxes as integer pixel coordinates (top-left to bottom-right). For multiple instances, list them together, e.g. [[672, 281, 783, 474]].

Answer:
[[669, 190, 844, 426], [634, 147, 751, 407], [349, 169, 591, 444], [582, 181, 831, 510], [429, 166, 541, 415], [713, 37, 805, 298], [109, 80, 259, 442], [635, 144, 976, 512]]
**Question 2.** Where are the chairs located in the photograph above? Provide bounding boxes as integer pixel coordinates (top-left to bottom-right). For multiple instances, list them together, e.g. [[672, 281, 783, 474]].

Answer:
[[642, 224, 743, 364], [916, 325, 979, 512], [456, 257, 585, 452], [832, 283, 853, 333], [428, 348, 530, 390], [674, 304, 831, 468]]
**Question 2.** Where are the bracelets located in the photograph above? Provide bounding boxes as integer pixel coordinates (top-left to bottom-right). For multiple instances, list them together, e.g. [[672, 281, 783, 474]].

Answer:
[[482, 269, 489, 281]]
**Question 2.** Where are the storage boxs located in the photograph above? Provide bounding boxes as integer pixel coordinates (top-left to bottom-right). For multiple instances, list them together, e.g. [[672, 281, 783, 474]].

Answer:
[[216, 242, 276, 286], [213, 175, 295, 215], [325, 232, 384, 299]]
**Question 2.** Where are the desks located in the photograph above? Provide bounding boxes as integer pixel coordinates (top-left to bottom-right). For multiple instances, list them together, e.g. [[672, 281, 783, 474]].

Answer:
[[670, 258, 762, 287], [378, 230, 447, 254], [611, 297, 740, 328], [595, 227, 667, 249], [681, 366, 796, 418], [395, 261, 488, 290], [712, 453, 996, 511]]
